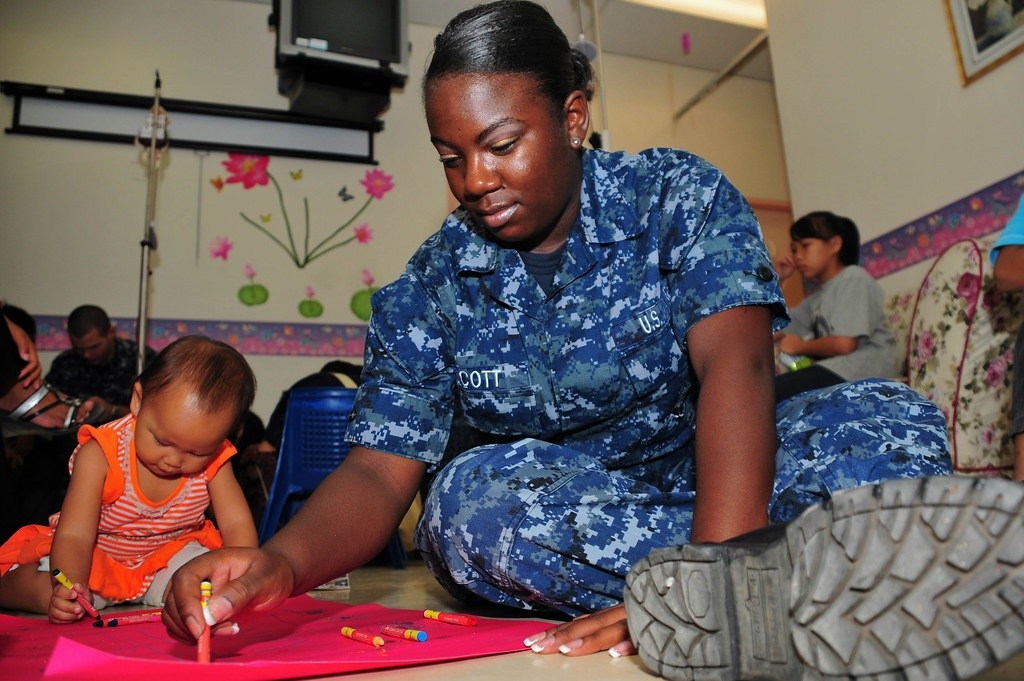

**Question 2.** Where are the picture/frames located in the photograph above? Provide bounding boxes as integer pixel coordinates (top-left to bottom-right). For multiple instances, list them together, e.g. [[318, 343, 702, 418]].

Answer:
[[942, 0, 1023, 87]]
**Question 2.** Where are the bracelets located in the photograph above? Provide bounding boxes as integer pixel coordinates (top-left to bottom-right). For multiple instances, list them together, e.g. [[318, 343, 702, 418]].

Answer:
[[111, 406, 117, 415]]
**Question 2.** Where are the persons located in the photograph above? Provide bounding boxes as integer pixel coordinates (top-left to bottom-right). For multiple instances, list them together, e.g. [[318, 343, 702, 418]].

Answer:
[[265, 356, 370, 464], [0, 335, 260, 625], [986, 184, 1024, 480], [40, 304, 159, 419], [0, 295, 108, 428], [159, 0, 1024, 657], [770, 210, 897, 399]]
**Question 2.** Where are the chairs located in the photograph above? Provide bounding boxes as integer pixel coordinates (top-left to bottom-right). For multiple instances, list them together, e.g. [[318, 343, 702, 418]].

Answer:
[[259, 386, 408, 567]]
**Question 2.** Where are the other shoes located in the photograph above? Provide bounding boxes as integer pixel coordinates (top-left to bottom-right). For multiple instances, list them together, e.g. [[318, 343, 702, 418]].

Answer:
[[623, 476, 1023, 681]]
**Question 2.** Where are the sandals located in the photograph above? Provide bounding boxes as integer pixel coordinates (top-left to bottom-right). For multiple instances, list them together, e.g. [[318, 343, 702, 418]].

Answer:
[[1, 383, 104, 431]]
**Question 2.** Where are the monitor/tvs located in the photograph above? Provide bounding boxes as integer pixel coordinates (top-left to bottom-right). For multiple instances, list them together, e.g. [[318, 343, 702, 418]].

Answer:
[[278, 0, 409, 77]]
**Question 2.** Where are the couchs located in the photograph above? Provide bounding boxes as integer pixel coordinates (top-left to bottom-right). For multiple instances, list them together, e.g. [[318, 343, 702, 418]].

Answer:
[[878, 238, 1014, 478]]
